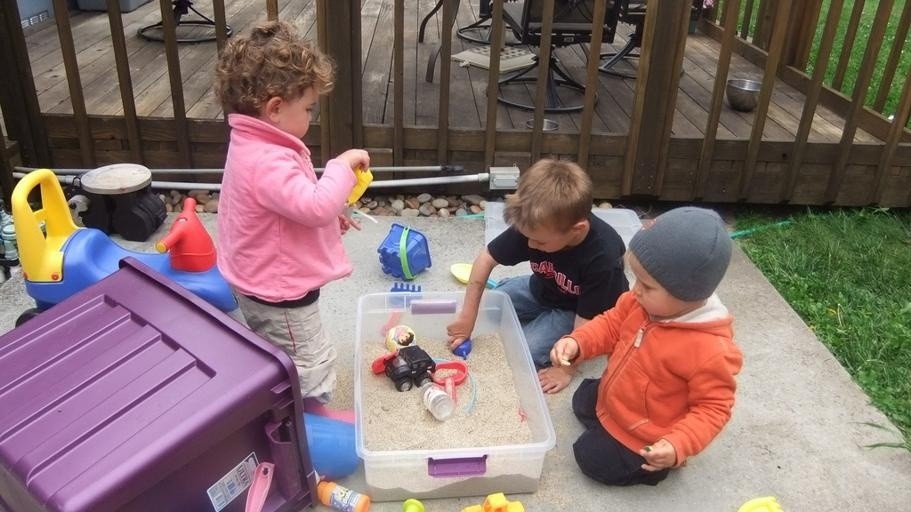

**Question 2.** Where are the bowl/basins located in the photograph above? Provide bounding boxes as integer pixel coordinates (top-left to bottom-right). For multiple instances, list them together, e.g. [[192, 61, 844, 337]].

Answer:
[[521, 120, 559, 135], [725, 78, 768, 111]]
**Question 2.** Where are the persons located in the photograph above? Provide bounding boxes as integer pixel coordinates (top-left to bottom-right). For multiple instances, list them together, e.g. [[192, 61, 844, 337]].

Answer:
[[549, 206, 743, 485], [446, 158, 626, 394], [216, 23, 370, 423]]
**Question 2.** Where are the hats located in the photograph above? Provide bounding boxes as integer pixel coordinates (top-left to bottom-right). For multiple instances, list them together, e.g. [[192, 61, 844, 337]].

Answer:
[[628, 206, 732, 302]]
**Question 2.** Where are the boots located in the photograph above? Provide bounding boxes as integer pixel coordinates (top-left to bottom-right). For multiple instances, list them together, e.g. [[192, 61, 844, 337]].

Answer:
[[303, 399, 355, 424]]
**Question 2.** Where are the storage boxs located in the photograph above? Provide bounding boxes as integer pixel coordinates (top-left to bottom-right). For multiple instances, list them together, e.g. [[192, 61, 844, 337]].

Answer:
[[355, 286, 558, 503], [0, 254, 317, 512]]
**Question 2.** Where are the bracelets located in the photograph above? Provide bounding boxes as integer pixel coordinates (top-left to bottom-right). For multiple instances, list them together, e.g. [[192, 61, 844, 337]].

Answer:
[[468, 279, 486, 288]]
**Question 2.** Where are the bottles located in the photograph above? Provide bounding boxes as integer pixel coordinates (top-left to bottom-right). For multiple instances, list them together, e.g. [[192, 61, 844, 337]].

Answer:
[[312, 480, 369, 511], [414, 377, 456, 422]]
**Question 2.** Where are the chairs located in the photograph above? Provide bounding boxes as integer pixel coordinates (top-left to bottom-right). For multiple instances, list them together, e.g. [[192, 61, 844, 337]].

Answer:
[[485, 0, 702, 116]]
